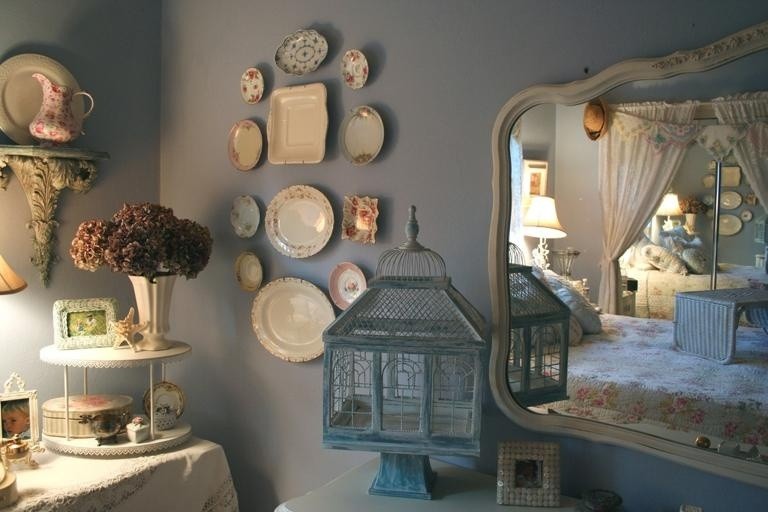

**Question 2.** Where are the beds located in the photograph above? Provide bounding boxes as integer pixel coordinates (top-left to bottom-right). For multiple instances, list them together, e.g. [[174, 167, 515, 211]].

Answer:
[[621, 229, 767, 327], [509, 311, 766, 466]]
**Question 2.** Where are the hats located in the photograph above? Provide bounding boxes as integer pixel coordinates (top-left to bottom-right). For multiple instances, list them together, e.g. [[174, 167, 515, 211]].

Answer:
[[583, 98, 610, 140]]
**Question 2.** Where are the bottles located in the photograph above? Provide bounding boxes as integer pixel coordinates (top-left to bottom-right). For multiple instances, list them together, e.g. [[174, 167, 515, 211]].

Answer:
[[124, 415, 149, 443]]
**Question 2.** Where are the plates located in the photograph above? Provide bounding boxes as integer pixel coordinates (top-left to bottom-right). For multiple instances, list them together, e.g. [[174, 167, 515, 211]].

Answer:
[[249, 280, 342, 361], [267, 83, 329, 169], [704, 158, 759, 238], [223, 119, 264, 173], [262, 182, 339, 262], [272, 29, 330, 76], [238, 69, 266, 106], [229, 196, 261, 240], [0, 51, 85, 147], [339, 109, 385, 164], [337, 49, 373, 94], [232, 253, 264, 290], [327, 264, 370, 312], [343, 194, 380, 246], [139, 382, 184, 423]]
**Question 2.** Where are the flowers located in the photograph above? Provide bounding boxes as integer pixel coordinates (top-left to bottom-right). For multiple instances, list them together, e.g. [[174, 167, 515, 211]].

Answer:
[[69, 199, 211, 286], [678, 196, 708, 215]]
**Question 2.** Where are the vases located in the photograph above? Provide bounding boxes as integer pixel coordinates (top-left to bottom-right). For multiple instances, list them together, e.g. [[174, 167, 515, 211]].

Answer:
[[129, 269, 178, 352], [686, 212, 697, 233]]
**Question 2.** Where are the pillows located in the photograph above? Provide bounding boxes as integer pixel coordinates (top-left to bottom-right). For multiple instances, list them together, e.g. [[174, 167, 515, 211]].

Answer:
[[630, 236, 713, 276], [507, 240, 602, 346]]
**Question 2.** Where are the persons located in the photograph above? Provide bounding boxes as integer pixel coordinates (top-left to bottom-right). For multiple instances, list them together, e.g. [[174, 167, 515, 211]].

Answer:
[[2, 401, 31, 440], [86, 314, 96, 327], [518, 465, 535, 487]]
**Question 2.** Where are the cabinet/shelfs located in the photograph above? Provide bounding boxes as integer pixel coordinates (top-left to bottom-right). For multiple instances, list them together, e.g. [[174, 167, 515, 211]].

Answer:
[[32, 339, 196, 467]]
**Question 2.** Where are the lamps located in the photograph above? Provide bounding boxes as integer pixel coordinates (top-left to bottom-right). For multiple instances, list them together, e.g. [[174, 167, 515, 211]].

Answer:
[[518, 189, 565, 270], [656, 188, 686, 229]]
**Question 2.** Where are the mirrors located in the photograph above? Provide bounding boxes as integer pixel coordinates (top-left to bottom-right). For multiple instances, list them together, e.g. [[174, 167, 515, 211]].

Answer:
[[486, 27, 766, 491]]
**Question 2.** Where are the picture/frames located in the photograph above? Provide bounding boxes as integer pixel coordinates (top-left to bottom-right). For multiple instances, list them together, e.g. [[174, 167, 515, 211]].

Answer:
[[52, 297, 123, 352], [1, 371, 43, 444], [492, 436, 562, 508]]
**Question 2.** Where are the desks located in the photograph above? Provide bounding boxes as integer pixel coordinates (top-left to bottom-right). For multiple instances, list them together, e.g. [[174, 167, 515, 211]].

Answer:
[[1, 419, 246, 510], [270, 450, 594, 510], [673, 285, 767, 361]]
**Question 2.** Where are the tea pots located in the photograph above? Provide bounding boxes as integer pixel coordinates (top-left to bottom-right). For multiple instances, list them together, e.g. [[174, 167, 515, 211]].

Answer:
[[28, 67, 93, 149]]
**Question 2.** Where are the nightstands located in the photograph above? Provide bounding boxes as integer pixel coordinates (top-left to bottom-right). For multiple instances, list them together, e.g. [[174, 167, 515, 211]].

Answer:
[[618, 290, 636, 318]]
